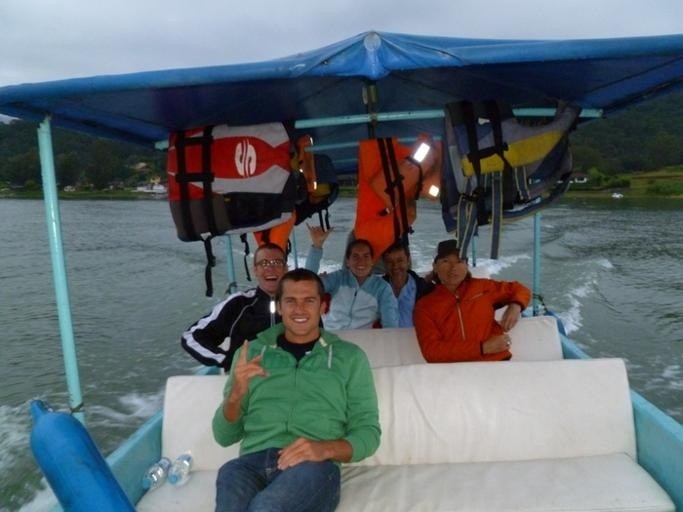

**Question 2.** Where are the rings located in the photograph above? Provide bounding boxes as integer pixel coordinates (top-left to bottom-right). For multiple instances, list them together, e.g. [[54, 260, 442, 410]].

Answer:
[[505, 339, 511, 348]]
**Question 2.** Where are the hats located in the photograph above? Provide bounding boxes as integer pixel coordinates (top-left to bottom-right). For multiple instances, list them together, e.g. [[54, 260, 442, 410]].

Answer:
[[435, 239, 468, 259]]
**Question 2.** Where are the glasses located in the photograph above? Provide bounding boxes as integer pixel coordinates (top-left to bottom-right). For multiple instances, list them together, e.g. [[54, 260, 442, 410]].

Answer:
[[255, 258, 285, 267]]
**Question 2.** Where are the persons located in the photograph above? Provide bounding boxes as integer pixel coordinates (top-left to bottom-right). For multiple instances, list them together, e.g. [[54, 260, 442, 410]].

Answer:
[[178, 241, 291, 374], [304, 220, 398, 330], [411, 239, 531, 366], [317, 242, 432, 330], [209, 268, 381, 512]]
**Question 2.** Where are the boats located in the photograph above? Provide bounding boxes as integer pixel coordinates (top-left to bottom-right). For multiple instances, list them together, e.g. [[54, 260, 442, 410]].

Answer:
[[0, 26, 682, 512]]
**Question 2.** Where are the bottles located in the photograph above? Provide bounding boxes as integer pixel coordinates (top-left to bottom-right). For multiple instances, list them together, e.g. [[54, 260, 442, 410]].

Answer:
[[167, 450, 193, 485], [140, 458, 170, 491]]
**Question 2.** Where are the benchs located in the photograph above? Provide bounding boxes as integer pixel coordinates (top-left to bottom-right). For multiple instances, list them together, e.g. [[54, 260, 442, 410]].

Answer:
[[325, 315, 566, 366], [133, 354, 676, 511]]
